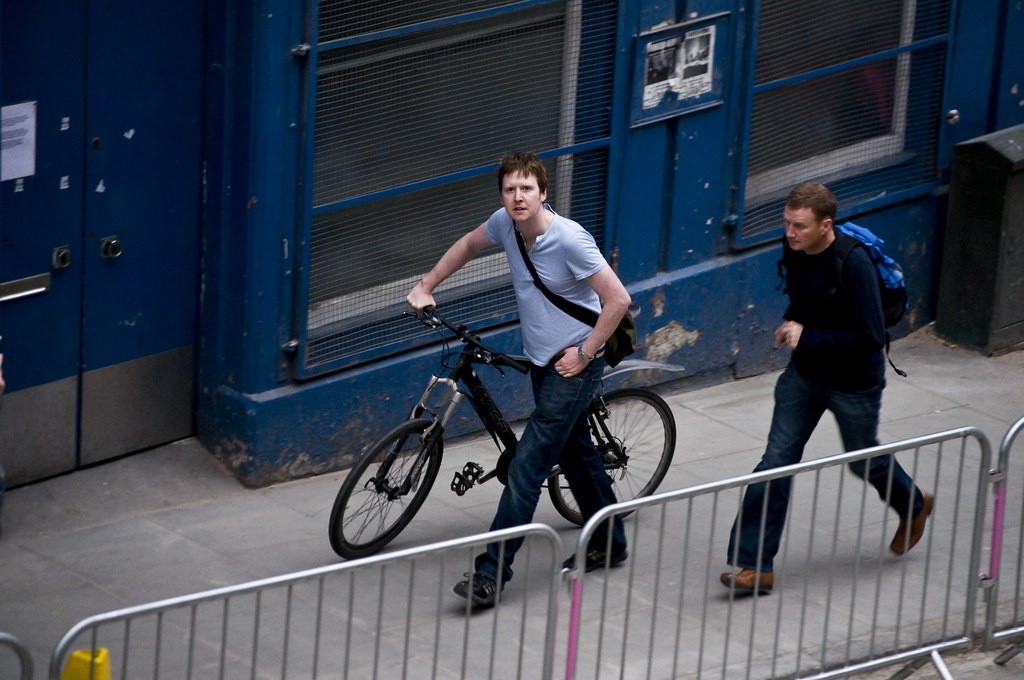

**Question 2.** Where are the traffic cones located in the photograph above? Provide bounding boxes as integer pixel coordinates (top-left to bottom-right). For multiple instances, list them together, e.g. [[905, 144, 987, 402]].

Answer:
[[56, 644, 117, 680]]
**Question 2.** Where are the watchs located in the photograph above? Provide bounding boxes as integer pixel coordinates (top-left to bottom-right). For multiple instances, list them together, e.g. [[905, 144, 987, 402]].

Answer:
[[577, 345, 594, 364]]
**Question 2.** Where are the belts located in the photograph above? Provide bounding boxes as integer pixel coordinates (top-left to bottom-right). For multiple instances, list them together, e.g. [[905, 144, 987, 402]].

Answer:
[[596, 349, 606, 359]]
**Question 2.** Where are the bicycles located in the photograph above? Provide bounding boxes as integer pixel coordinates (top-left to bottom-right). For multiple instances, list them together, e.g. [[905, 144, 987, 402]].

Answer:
[[326, 307, 688, 560]]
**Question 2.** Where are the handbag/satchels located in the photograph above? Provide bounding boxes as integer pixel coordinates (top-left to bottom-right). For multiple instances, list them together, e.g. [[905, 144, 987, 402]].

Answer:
[[599, 295, 638, 368]]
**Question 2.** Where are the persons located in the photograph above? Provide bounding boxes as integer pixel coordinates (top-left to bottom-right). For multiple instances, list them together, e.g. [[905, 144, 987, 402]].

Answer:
[[721, 180, 936, 592], [406, 147, 632, 608]]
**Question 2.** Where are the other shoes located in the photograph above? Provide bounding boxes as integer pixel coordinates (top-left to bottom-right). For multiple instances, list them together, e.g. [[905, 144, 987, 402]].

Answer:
[[888, 494, 934, 556], [720, 567, 773, 594]]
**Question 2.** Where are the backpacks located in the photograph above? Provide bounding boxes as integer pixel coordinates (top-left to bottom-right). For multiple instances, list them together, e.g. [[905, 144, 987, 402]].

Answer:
[[783, 220, 908, 329]]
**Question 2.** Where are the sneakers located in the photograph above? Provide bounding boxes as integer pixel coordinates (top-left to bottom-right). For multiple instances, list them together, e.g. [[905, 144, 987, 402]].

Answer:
[[454, 571, 504, 604], [562, 545, 629, 574]]
